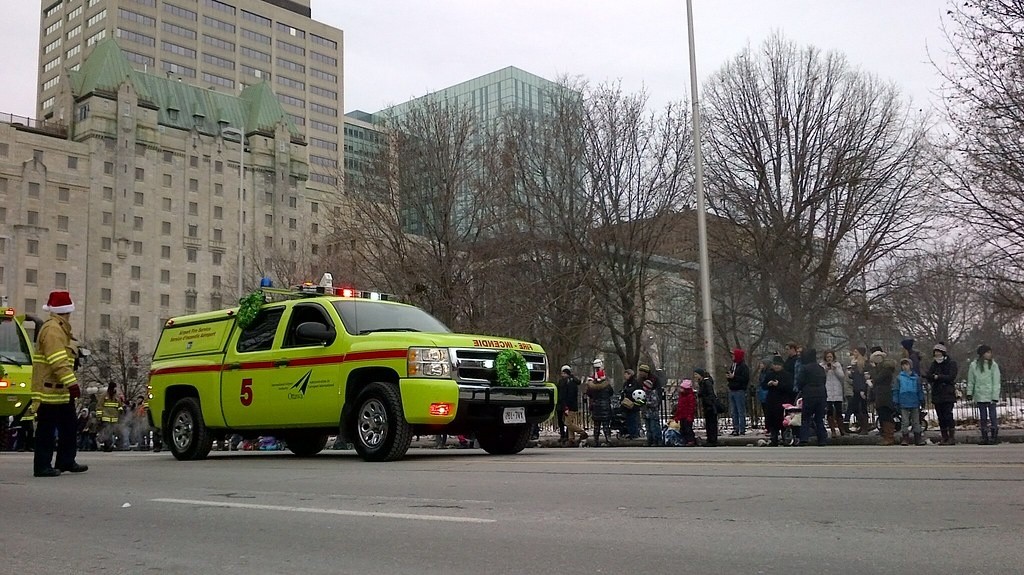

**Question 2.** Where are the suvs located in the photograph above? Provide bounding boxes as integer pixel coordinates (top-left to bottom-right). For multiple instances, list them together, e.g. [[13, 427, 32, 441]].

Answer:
[[145, 273, 558, 461]]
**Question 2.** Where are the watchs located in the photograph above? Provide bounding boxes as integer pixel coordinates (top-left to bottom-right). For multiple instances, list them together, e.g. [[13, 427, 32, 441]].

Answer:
[[932, 374, 935, 379]]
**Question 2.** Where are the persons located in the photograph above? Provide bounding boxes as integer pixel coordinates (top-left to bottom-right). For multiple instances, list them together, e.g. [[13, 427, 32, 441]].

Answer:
[[921, 341, 958, 445], [967, 345, 1001, 445], [31, 291, 89, 477], [18, 380, 169, 452], [555, 359, 697, 447], [893, 358, 926, 445], [694, 369, 718, 447], [726, 350, 750, 437], [758, 339, 923, 447]]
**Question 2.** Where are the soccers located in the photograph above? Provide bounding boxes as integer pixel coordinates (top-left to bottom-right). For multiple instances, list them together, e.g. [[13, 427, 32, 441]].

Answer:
[[632, 389, 646, 402]]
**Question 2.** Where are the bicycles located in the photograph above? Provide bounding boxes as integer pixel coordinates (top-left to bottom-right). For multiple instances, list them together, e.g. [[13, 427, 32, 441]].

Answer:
[[876, 415, 928, 433]]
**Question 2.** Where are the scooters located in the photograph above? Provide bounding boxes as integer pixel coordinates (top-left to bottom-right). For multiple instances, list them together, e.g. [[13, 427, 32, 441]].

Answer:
[[611, 399, 645, 441]]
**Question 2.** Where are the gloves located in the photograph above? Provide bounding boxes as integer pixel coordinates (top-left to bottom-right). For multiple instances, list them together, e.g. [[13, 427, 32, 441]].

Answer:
[[564, 406, 569, 415], [634, 402, 640, 406], [967, 395, 972, 401], [68, 384, 80, 398], [638, 399, 645, 406]]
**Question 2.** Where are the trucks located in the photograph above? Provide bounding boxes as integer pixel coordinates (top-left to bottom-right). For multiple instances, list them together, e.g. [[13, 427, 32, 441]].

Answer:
[[0, 296, 44, 452]]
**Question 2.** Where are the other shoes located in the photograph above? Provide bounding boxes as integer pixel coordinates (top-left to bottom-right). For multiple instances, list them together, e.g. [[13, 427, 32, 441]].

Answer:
[[55, 461, 88, 472], [558, 431, 747, 447], [34, 468, 61, 476], [766, 431, 825, 447]]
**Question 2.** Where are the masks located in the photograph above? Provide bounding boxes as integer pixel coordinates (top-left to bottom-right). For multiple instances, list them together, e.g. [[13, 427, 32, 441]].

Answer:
[[874, 356, 883, 363]]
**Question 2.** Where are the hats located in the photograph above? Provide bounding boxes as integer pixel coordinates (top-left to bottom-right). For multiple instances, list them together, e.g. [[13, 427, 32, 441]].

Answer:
[[681, 380, 692, 389], [42, 290, 75, 314], [977, 345, 992, 355], [772, 356, 784, 367], [597, 365, 656, 389], [870, 346, 886, 360], [856, 347, 866, 356], [901, 338, 913, 351], [933, 340, 946, 351], [694, 368, 704, 376], [900, 358, 913, 369]]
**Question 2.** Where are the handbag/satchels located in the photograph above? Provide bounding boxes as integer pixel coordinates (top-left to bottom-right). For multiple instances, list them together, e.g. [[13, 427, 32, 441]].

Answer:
[[621, 398, 633, 409], [760, 386, 770, 406], [715, 398, 726, 413]]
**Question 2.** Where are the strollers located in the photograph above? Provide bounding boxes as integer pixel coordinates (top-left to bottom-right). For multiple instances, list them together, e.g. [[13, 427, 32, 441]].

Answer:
[[660, 406, 687, 446], [782, 390, 818, 447]]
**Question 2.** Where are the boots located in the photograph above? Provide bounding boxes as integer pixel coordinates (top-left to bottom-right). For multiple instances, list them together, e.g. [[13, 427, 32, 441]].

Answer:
[[827, 414, 999, 445]]
